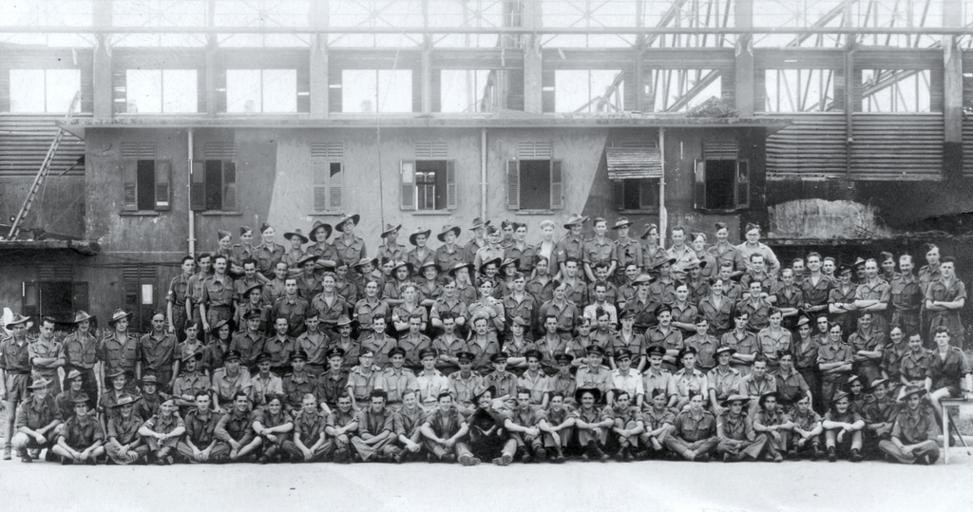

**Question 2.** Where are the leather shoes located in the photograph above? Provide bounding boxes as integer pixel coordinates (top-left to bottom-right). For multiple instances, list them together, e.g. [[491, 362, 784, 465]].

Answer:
[[917, 453, 929, 465], [883, 452, 896, 463], [847, 448, 863, 462], [827, 446, 838, 462]]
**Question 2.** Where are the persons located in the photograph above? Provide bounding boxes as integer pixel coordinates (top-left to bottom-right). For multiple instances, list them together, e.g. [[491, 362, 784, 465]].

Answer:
[[1, 215, 973, 465]]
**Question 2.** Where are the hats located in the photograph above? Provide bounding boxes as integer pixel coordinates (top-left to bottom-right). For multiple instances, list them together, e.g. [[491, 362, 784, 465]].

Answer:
[[524, 344, 634, 364], [833, 371, 927, 404], [653, 255, 676, 269], [242, 281, 359, 329], [218, 221, 269, 243], [688, 389, 780, 406], [574, 383, 666, 402], [444, 272, 525, 286], [563, 212, 590, 228], [833, 250, 895, 273], [690, 223, 759, 241], [683, 259, 707, 272], [472, 382, 496, 399], [284, 214, 361, 242], [792, 311, 842, 329], [409, 226, 431, 245], [609, 217, 632, 228], [381, 222, 402, 238], [387, 346, 510, 362], [645, 343, 738, 357], [182, 317, 344, 362], [486, 219, 524, 232], [468, 216, 490, 229], [5, 306, 132, 330], [296, 252, 379, 274], [438, 223, 463, 242], [639, 222, 657, 239], [574, 303, 673, 319], [508, 313, 529, 329], [24, 367, 162, 407], [382, 255, 521, 272], [631, 270, 654, 285], [675, 274, 778, 324], [535, 255, 548, 264]]
